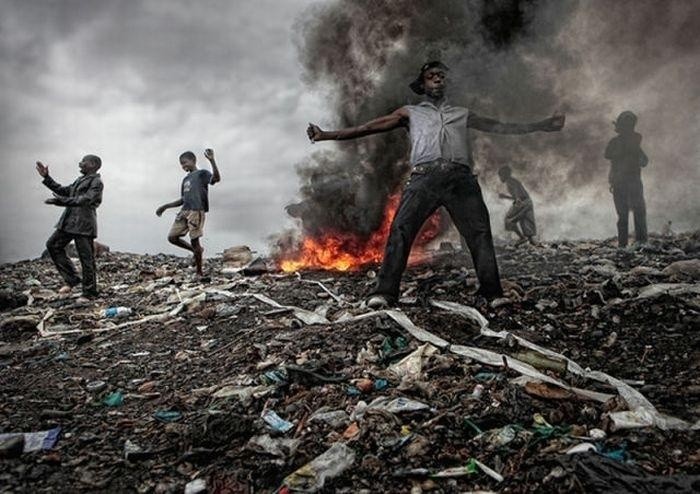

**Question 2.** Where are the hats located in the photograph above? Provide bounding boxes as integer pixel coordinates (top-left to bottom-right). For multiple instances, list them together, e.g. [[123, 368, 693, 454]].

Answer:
[[407, 59, 451, 95]]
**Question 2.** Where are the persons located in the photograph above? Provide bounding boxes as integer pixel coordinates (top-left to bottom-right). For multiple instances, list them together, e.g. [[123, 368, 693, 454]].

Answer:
[[603, 110, 649, 246], [306, 59, 565, 310], [155, 147, 221, 282], [35, 153, 104, 302], [498, 165, 537, 246]]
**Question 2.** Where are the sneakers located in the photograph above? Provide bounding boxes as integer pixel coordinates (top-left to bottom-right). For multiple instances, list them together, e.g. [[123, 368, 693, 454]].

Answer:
[[488, 296, 512, 309], [366, 295, 388, 308], [75, 295, 91, 304], [191, 248, 204, 267], [58, 285, 71, 293]]
